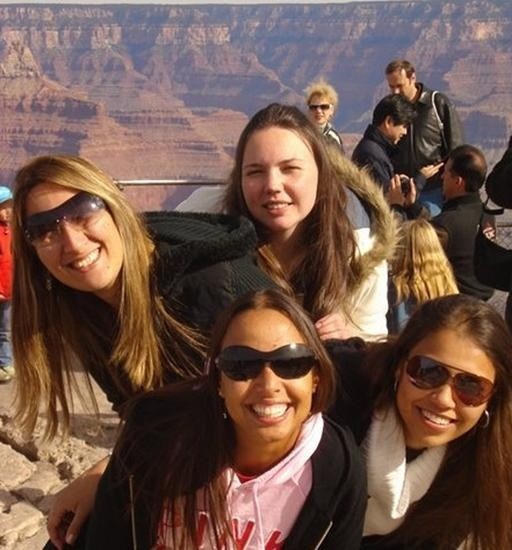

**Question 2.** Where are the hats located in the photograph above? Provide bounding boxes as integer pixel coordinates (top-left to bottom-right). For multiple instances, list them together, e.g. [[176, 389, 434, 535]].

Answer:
[[0, 186, 13, 204]]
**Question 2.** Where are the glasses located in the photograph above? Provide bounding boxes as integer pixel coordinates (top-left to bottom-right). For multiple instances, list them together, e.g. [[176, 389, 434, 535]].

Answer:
[[217, 342, 316, 381], [404, 354, 496, 407], [309, 104, 330, 111], [23, 190, 105, 242]]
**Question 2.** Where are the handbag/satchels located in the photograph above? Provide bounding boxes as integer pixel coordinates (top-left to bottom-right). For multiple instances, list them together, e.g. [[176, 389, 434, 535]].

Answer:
[[471, 195, 512, 294]]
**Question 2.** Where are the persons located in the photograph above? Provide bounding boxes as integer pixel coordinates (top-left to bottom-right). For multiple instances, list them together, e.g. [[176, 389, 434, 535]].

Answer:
[[0, 186, 15, 381], [46, 294, 512, 550], [303, 79, 346, 155], [83, 288, 368, 550], [12, 155, 293, 446], [352, 95, 443, 210], [386, 59, 464, 219], [387, 218, 460, 335], [213, 102, 403, 343], [387, 145, 496, 302]]
